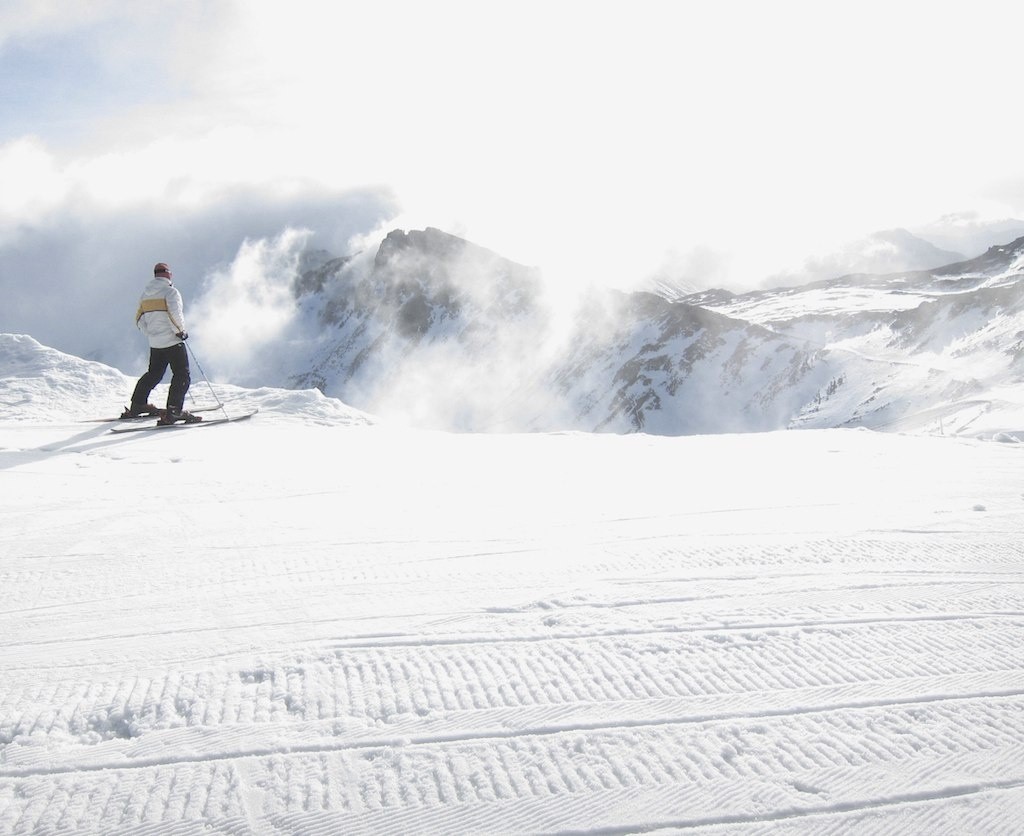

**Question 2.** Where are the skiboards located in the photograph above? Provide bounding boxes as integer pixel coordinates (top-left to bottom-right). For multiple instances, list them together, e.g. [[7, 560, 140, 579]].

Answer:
[[72, 401, 260, 434]]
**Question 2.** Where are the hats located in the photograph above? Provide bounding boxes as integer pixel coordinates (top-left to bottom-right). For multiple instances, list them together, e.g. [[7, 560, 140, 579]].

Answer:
[[154, 263, 172, 278]]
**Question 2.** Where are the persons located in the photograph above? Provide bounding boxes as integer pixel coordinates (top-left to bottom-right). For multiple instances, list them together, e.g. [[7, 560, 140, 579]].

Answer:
[[131, 263, 197, 420]]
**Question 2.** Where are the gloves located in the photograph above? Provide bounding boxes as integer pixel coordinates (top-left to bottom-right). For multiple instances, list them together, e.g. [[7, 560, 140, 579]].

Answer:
[[176, 332, 188, 340]]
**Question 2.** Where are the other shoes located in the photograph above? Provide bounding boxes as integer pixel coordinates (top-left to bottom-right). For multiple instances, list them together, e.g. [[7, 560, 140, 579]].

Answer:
[[166, 406, 195, 420], [130, 401, 157, 412]]
